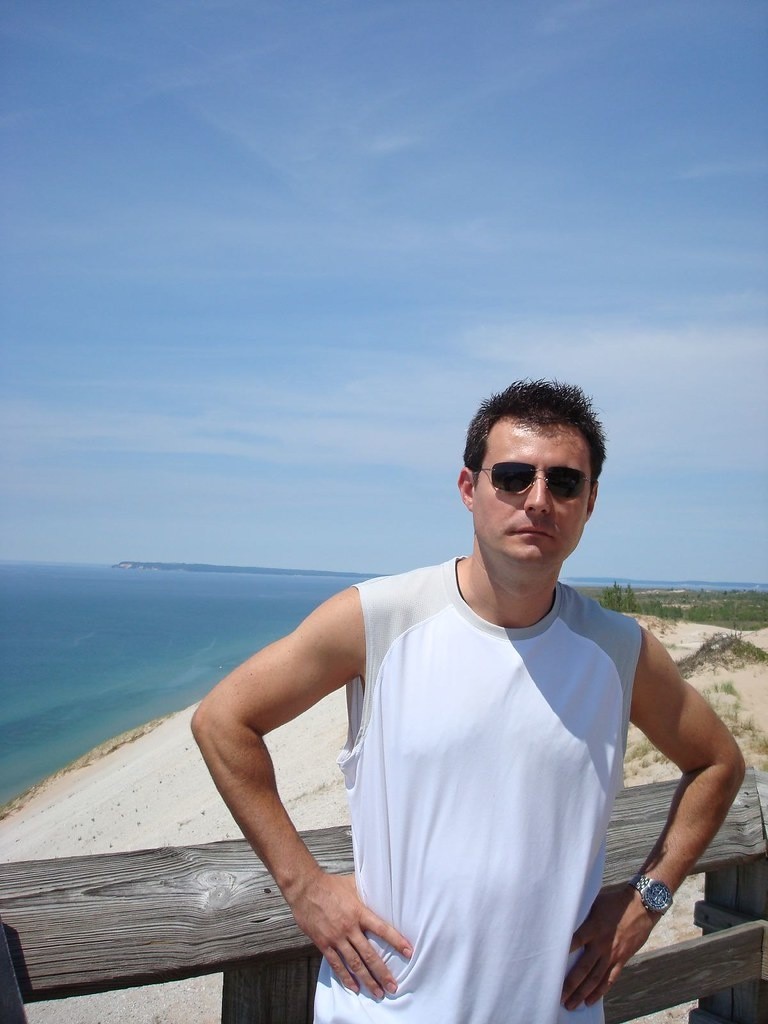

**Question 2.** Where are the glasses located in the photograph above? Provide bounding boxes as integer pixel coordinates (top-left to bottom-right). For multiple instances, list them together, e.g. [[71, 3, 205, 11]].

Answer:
[[478, 461, 593, 498]]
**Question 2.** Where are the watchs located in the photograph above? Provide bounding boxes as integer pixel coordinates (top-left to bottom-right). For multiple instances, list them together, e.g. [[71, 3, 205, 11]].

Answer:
[[628, 873, 673, 916]]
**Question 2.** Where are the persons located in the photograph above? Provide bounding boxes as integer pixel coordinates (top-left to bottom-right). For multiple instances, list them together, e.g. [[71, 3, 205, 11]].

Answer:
[[193, 378, 746, 1024]]
